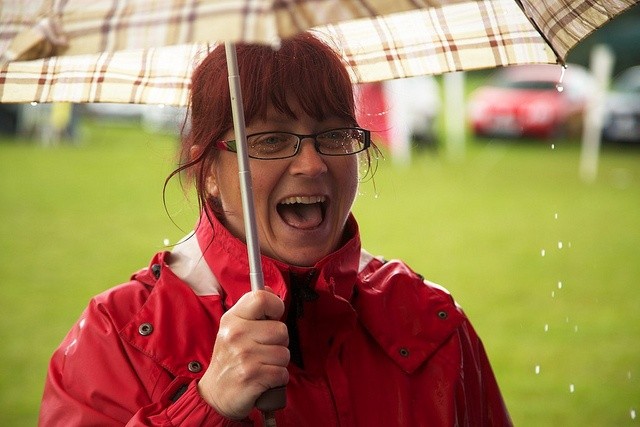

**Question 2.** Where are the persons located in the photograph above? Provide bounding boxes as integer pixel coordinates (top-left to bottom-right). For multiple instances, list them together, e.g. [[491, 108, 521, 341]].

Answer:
[[35, 31, 516, 426]]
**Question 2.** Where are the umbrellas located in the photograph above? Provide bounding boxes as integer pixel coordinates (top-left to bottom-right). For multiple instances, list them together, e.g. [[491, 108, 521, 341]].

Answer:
[[0, 0, 638, 410]]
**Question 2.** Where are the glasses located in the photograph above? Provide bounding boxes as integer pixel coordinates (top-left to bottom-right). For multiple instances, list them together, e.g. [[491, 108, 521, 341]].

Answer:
[[212, 128, 371, 160]]
